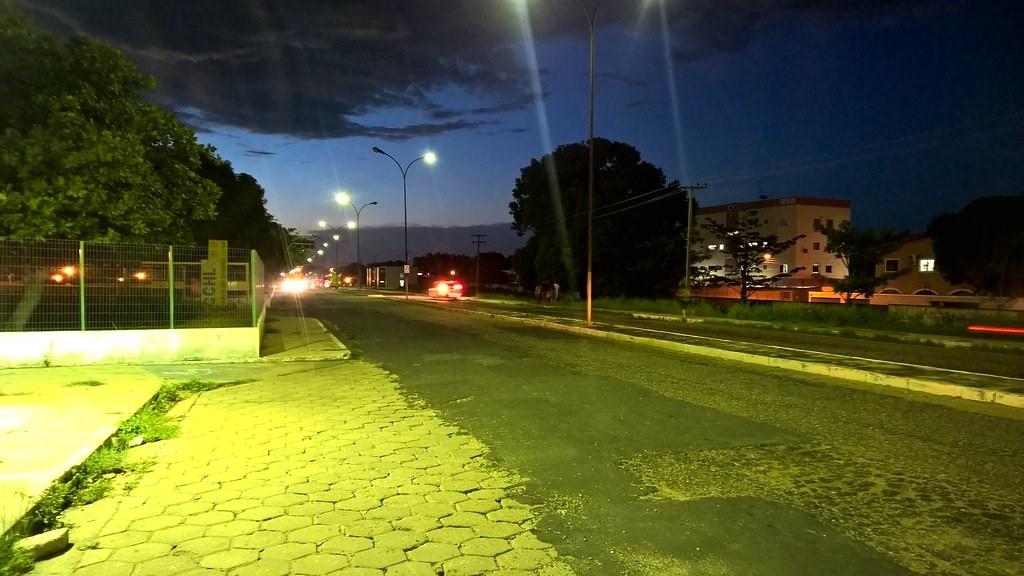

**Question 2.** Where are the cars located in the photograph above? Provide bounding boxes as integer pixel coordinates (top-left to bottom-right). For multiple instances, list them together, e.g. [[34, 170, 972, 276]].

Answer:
[[427, 281, 463, 300], [282, 272, 360, 292]]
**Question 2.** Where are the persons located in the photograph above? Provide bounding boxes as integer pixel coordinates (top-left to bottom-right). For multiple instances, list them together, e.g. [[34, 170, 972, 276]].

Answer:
[[551, 280, 559, 304], [534, 283, 547, 304], [9, 272, 15, 288]]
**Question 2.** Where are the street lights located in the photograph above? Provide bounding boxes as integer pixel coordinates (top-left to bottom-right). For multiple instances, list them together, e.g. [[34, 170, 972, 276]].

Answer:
[[336, 192, 378, 290], [307, 234, 339, 274], [372, 146, 435, 301], [319, 221, 355, 291]]
